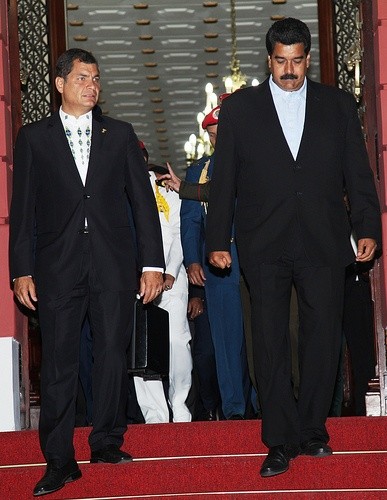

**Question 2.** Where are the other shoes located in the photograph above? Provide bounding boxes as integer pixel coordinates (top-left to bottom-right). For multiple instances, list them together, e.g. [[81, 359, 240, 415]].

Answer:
[[172, 403, 192, 423], [229, 414, 244, 420]]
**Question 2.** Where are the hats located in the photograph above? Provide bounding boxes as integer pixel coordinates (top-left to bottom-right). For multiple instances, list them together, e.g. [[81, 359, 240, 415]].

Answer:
[[202, 104, 222, 129], [139, 141, 148, 163]]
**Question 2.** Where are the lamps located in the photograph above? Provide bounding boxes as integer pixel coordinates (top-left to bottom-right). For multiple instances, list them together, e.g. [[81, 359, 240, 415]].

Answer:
[[185, 0, 260, 168]]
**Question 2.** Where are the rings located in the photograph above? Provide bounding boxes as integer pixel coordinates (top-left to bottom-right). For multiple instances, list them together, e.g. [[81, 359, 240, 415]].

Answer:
[[156, 290, 160, 292], [199, 310, 201, 312], [166, 285, 170, 289]]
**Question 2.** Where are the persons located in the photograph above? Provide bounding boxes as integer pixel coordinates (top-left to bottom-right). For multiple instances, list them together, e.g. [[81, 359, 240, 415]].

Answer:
[[9, 49, 165, 496], [74, 93, 374, 427], [203, 17, 382, 476]]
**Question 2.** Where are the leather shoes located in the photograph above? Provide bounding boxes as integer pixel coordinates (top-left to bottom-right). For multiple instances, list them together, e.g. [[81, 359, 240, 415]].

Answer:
[[260, 447, 298, 477], [32, 465, 83, 496], [90, 447, 133, 464], [300, 443, 332, 457]]
